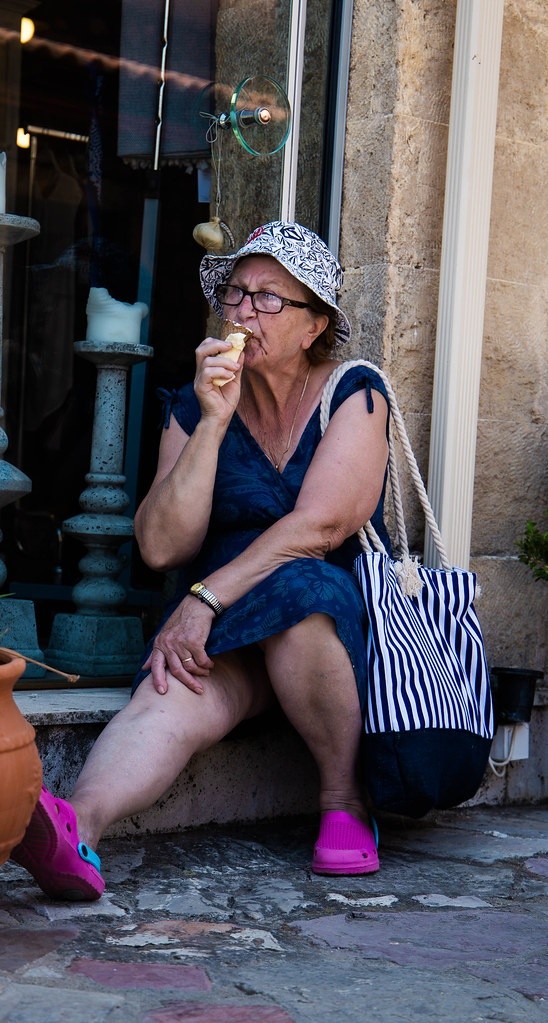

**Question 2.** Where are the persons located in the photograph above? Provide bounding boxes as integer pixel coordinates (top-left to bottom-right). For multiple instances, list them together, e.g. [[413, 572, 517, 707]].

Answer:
[[11, 220, 395, 900]]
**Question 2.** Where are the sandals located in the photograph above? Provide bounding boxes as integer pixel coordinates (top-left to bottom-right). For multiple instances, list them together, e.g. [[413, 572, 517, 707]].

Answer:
[[8, 785, 107, 901], [311, 803, 379, 875]]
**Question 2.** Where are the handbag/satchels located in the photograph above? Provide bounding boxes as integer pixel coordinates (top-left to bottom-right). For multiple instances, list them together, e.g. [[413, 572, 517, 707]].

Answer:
[[319, 356, 493, 818]]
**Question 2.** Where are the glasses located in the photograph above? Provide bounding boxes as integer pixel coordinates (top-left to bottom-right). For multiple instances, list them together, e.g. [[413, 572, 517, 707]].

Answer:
[[215, 283, 312, 315]]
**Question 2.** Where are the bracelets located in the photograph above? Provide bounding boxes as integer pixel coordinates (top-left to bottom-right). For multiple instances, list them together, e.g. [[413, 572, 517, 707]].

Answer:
[[190, 592, 220, 618]]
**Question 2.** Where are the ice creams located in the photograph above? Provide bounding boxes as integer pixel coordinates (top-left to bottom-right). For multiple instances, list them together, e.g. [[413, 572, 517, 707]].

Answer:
[[218, 319, 254, 347]]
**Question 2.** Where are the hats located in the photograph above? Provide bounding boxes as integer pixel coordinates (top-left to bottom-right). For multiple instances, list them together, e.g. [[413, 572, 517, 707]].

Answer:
[[198, 221, 351, 347]]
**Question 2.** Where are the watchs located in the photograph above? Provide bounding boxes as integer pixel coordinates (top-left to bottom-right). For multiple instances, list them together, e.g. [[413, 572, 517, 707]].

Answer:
[[190, 581, 223, 615]]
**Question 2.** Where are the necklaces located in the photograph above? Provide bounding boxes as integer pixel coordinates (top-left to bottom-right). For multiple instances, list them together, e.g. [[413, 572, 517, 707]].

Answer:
[[241, 363, 312, 469]]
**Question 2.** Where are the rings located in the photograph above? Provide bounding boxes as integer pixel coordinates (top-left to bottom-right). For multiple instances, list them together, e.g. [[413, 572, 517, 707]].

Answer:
[[182, 657, 192, 663]]
